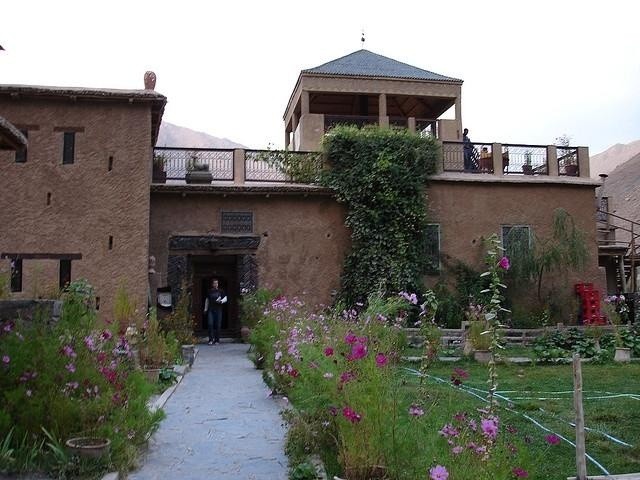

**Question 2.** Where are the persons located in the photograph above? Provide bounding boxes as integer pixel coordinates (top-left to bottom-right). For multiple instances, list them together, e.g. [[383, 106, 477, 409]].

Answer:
[[462, 128, 475, 172], [204, 279, 227, 345]]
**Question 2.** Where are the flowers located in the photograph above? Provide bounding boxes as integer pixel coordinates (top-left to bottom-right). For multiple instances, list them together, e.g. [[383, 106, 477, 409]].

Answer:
[[0, 256, 166, 479]]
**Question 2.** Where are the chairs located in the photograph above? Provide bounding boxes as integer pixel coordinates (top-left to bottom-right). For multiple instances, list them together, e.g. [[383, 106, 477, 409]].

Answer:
[[479, 150, 508, 174]]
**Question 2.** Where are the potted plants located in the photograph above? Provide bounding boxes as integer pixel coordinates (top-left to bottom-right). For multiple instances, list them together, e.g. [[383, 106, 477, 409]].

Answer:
[[522, 152, 532, 175], [471, 314, 494, 363], [171, 292, 195, 362], [555, 132, 577, 175], [610, 328, 635, 362], [153, 153, 167, 183]]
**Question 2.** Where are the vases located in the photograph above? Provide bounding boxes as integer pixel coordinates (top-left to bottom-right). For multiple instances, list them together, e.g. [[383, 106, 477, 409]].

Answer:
[[64, 436, 110, 462], [142, 368, 162, 386]]
